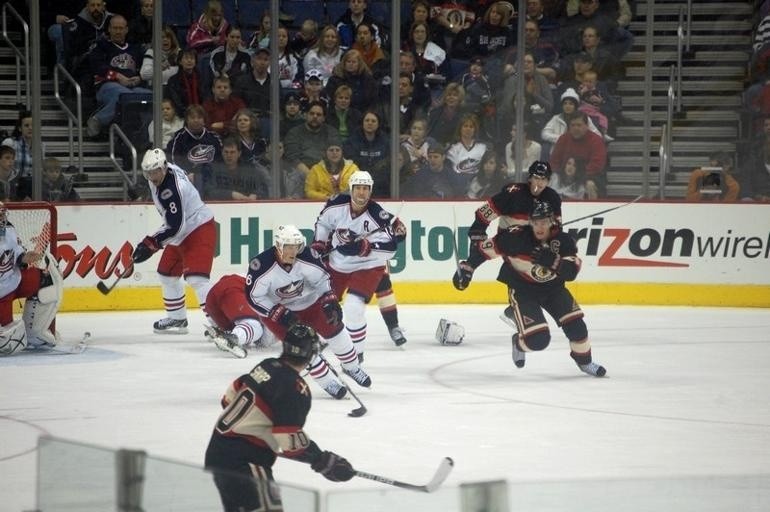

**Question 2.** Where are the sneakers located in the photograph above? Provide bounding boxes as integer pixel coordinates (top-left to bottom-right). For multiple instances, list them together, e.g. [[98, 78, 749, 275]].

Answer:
[[341, 365, 373, 392], [511, 333, 526, 369], [204, 326, 226, 340], [499, 305, 518, 330], [574, 355, 606, 377], [214, 334, 248, 359], [322, 379, 347, 400], [388, 324, 408, 351], [152, 312, 189, 335]]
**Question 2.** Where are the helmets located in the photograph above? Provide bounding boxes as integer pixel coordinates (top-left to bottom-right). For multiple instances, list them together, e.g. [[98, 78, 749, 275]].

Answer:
[[140, 148, 168, 186], [272, 224, 307, 263], [0, 201, 8, 227], [529, 159, 552, 179], [529, 202, 556, 224], [348, 170, 374, 207], [280, 324, 320, 371]]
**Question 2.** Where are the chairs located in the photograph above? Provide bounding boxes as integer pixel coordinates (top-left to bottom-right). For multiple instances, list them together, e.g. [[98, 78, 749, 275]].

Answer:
[[280, 1, 325, 32], [190, 0, 235, 28], [162, 0, 190, 42], [328, 0, 387, 25], [237, 0, 271, 29]]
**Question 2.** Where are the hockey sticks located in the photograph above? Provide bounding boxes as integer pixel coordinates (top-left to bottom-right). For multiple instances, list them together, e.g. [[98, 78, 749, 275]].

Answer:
[[451, 207, 462, 289], [317, 355, 367, 416], [29, 331, 90, 356], [558, 195, 643, 229], [321, 199, 406, 259], [97, 258, 135, 294], [355, 456, 455, 495]]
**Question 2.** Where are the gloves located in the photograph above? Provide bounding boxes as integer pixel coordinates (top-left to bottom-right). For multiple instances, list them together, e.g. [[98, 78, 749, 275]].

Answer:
[[267, 303, 296, 327], [322, 298, 343, 327], [131, 235, 164, 263], [532, 244, 557, 268], [309, 449, 355, 484], [335, 239, 363, 258], [452, 262, 473, 290]]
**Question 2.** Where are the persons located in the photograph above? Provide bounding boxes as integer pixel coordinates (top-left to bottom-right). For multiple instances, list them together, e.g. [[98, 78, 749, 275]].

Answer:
[[0, 204, 60, 353], [746, 0, 770, 196], [688, 167, 740, 202], [453, 201, 606, 378], [467, 160, 563, 329], [245, 224, 371, 400], [1, 0, 634, 201], [320, 193, 406, 346], [132, 150, 219, 335], [204, 272, 277, 358], [204, 326, 357, 512], [311, 171, 397, 364]]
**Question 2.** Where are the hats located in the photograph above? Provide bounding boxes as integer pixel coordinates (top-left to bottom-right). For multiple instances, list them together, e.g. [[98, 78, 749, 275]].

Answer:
[[426, 142, 445, 156], [324, 135, 344, 150], [304, 68, 324, 83], [560, 87, 581, 105]]
